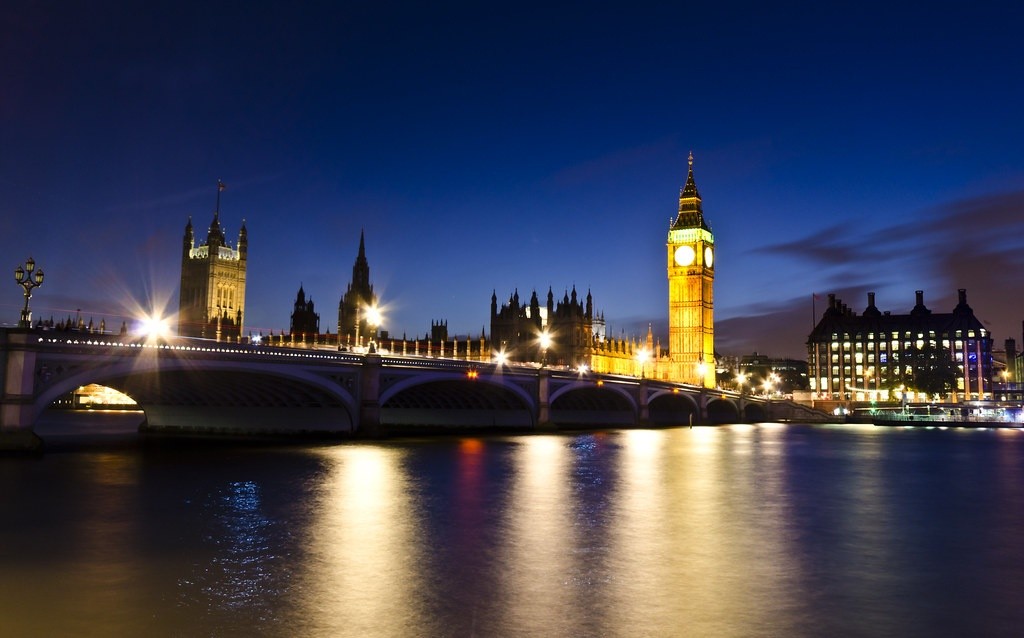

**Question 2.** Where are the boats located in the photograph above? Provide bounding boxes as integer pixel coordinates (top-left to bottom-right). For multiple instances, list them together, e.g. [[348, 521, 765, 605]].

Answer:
[[868, 404, 1024, 427]]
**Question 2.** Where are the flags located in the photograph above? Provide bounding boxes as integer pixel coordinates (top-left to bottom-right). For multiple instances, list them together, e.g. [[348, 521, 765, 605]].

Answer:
[[814, 294, 821, 301]]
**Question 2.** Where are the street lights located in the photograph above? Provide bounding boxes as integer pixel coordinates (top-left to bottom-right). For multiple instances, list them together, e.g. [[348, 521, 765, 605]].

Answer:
[[10, 258, 46, 330]]
